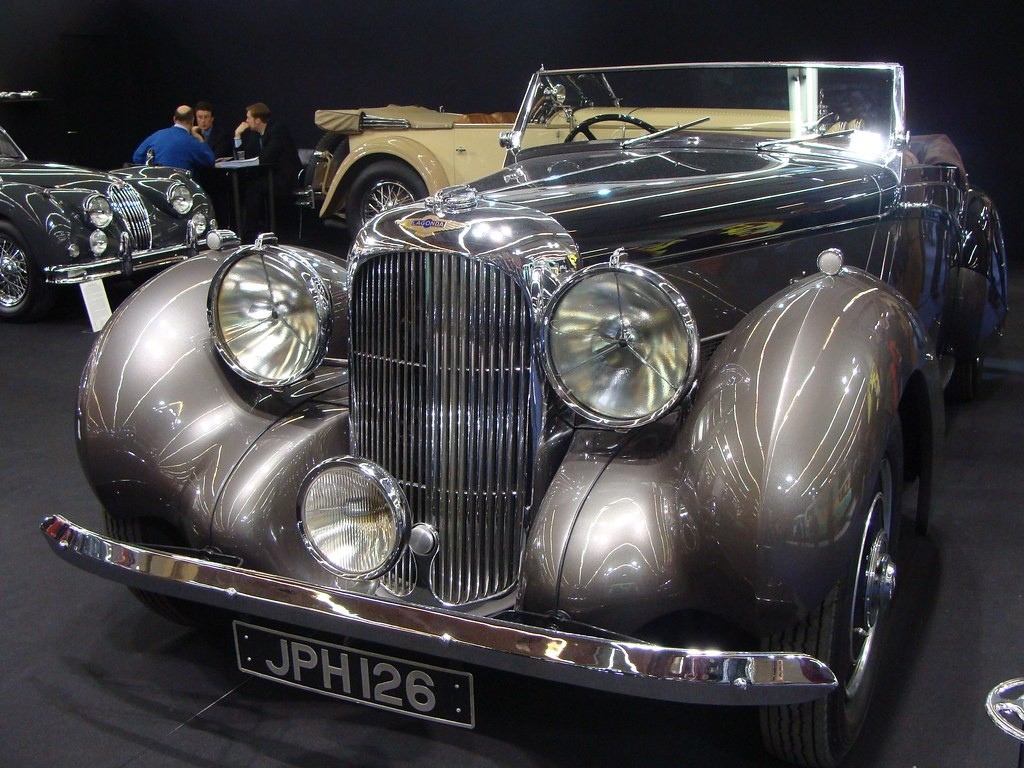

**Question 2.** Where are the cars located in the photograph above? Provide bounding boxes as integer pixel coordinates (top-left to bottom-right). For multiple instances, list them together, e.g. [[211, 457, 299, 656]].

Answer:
[[28, 58, 1010, 768], [0, 87, 217, 323], [289, 100, 968, 239]]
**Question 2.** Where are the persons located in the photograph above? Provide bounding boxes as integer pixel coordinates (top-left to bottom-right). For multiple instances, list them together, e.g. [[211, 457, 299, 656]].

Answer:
[[133, 105, 215, 177], [194, 101, 232, 159], [233, 102, 302, 233]]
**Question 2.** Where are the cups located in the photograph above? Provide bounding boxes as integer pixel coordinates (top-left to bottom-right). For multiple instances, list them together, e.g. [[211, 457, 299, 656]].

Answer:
[[237, 151, 245, 160]]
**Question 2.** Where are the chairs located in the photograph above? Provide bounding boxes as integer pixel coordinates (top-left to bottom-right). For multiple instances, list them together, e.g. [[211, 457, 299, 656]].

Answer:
[[289, 147, 315, 241], [491, 111, 517, 123], [457, 113, 498, 124]]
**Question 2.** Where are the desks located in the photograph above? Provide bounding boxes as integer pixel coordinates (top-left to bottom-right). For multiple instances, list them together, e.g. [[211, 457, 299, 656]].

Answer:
[[199, 162, 278, 244]]
